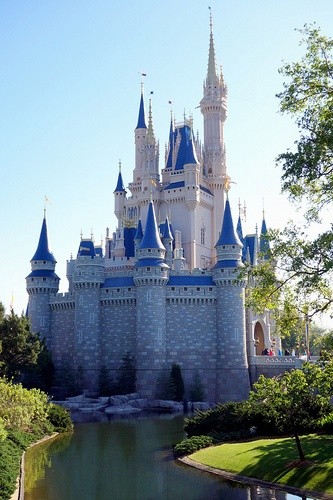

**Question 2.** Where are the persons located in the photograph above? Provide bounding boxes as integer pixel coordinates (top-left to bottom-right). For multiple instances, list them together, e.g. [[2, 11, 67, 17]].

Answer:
[[260, 347, 307, 356]]
